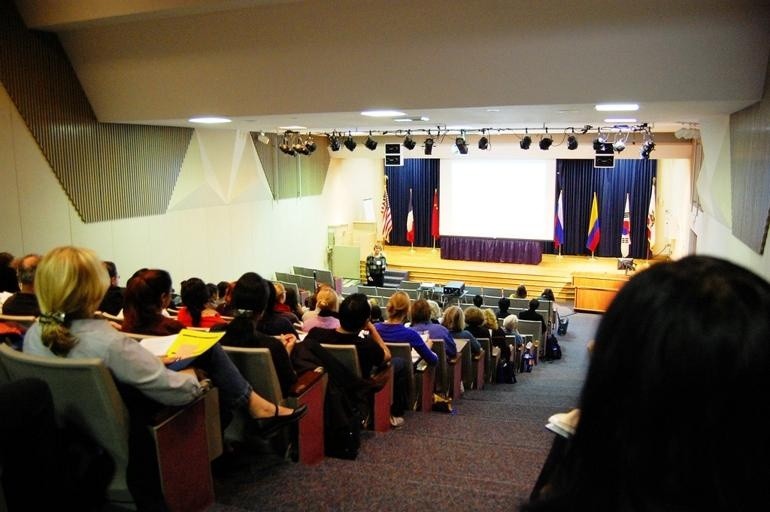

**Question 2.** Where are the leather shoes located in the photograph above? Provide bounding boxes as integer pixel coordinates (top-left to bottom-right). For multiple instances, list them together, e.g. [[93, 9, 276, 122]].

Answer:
[[247, 403, 310, 447], [356, 376, 388, 399]]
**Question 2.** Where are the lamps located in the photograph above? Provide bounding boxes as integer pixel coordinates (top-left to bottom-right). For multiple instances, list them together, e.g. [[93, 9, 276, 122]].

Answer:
[[278, 123, 656, 160], [674, 121, 700, 140], [257, 130, 269, 144]]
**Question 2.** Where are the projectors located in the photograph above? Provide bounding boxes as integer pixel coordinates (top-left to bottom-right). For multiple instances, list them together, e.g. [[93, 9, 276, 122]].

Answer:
[[444, 283, 464, 295]]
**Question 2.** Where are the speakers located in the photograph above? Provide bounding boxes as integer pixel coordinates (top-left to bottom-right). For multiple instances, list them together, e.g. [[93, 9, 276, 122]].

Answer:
[[593, 154, 616, 169], [592, 142, 616, 154], [385, 154, 405, 166], [384, 144, 403, 153]]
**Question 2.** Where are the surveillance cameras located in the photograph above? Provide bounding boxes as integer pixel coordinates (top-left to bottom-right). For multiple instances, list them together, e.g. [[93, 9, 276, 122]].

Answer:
[[256, 132, 269, 144]]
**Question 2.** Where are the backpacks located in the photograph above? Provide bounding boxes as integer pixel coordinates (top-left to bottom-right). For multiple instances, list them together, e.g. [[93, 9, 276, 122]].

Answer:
[[544, 334, 563, 362]]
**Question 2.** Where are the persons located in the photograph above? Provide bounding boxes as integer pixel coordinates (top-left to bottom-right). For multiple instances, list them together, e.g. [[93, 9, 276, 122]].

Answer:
[[1, 246, 387, 464], [532, 253, 770, 509], [306, 284, 557, 428]]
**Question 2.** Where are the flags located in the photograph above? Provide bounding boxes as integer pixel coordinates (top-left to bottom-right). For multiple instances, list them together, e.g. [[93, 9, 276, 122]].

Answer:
[[646, 185, 656, 249], [620, 194, 631, 256], [431, 193, 439, 241], [586, 195, 600, 252], [554, 191, 564, 249], [380, 186, 393, 244], [406, 192, 415, 244]]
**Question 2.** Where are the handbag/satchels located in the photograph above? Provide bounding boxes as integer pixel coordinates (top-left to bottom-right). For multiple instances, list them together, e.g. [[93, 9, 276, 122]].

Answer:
[[495, 358, 517, 385]]
